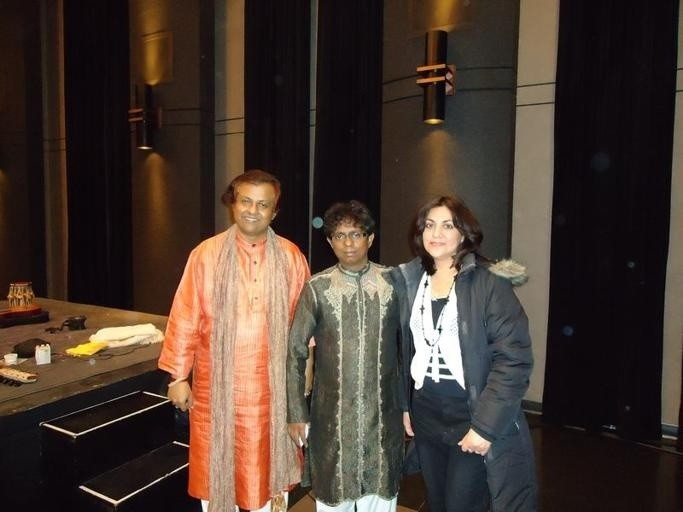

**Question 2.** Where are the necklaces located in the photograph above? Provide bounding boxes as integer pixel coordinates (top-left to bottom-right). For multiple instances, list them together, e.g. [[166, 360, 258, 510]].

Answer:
[[420, 271, 458, 349]]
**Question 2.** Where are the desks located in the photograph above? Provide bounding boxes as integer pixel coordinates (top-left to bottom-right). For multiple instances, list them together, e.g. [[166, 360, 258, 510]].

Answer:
[[0, 296, 169, 480]]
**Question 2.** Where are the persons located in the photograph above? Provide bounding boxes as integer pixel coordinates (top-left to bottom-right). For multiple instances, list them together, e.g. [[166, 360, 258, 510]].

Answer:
[[376, 196, 535, 510], [283, 197, 533, 512], [154, 167, 317, 512]]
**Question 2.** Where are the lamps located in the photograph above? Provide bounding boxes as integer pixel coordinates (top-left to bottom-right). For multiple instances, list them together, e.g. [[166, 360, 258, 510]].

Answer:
[[413, 28, 456, 127], [127, 81, 164, 153]]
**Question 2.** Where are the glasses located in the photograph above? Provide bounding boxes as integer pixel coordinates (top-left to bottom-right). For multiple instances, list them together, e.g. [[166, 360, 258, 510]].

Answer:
[[332, 230, 367, 241]]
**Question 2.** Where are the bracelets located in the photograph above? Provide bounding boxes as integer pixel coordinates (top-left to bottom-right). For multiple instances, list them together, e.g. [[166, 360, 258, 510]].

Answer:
[[166, 377, 191, 387]]
